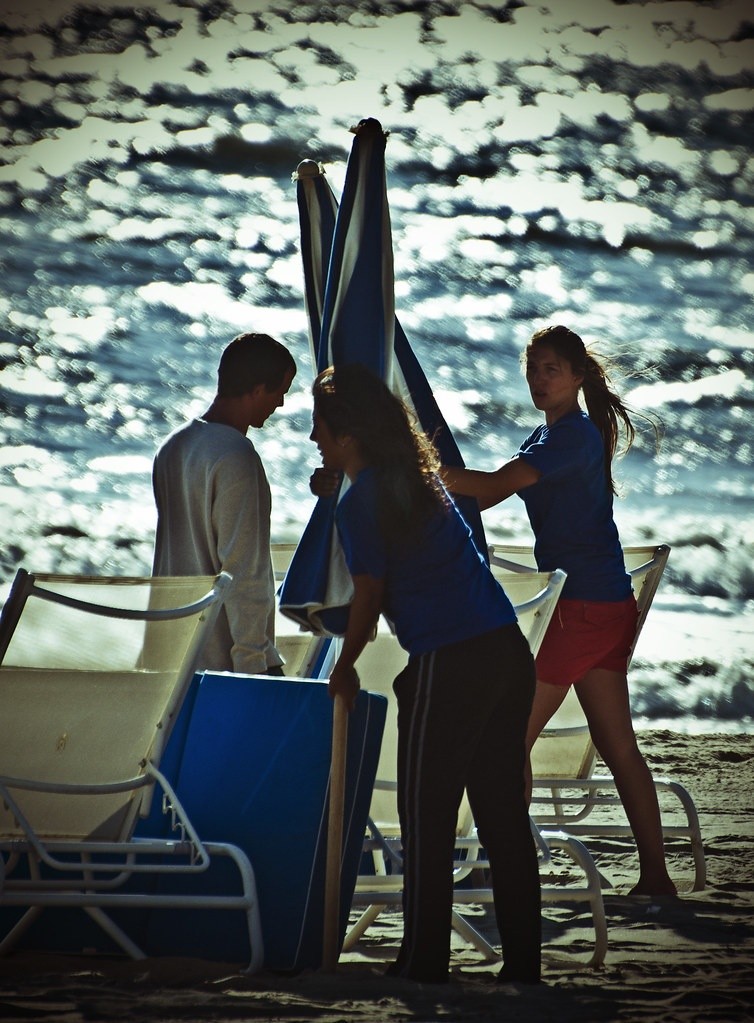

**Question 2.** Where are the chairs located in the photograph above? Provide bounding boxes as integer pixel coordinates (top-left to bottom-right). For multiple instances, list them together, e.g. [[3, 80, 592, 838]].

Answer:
[[269, 542, 706, 964], [0, 568, 266, 981]]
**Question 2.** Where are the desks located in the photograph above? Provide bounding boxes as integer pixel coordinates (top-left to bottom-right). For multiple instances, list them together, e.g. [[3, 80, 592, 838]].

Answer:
[[1, 674, 389, 975]]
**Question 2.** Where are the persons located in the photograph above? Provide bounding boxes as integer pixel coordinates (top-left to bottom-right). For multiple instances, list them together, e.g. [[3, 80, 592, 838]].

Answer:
[[134, 332, 297, 680], [308, 365, 542, 997], [428, 324, 678, 909]]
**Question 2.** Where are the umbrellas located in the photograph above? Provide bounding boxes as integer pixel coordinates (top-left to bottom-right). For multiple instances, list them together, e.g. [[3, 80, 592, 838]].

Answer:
[[278, 118, 493, 992]]
